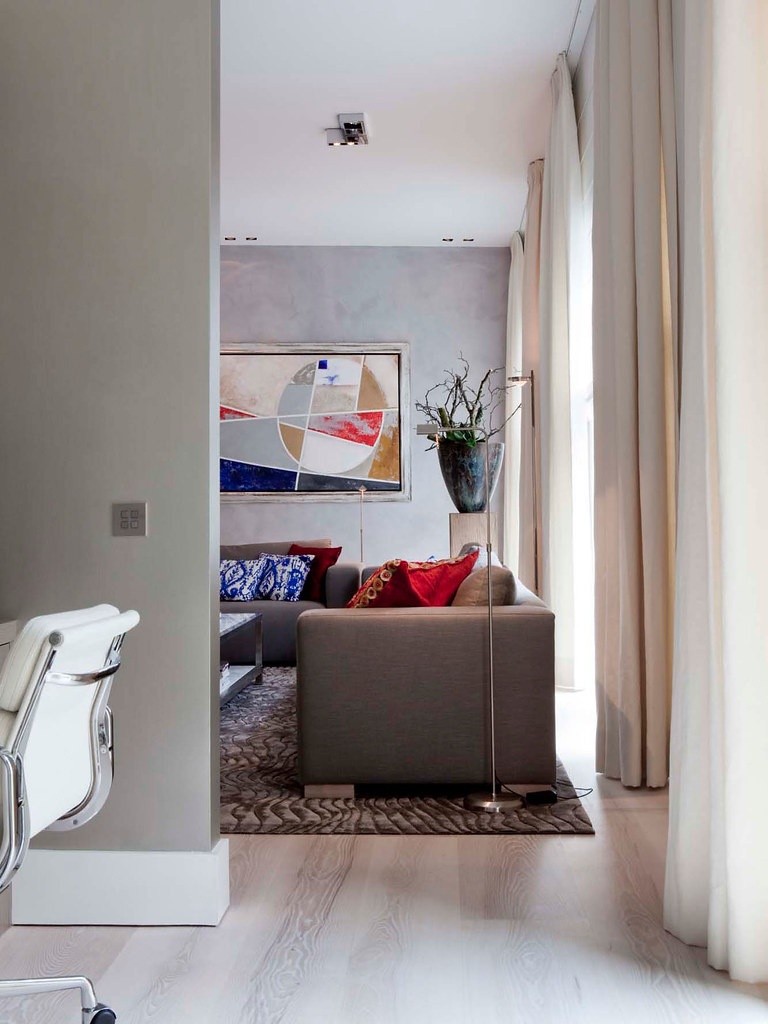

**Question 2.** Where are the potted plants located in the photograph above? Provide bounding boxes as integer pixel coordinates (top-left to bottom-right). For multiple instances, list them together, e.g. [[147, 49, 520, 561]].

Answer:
[[415, 350, 521, 514]]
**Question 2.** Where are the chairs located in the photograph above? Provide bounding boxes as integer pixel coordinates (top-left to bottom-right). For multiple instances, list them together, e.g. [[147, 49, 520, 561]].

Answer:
[[1, 603, 139, 1022]]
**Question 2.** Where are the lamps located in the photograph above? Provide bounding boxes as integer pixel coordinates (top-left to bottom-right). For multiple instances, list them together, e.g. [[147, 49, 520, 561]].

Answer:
[[358, 485, 367, 562], [416, 424, 524, 812], [507, 369, 540, 597]]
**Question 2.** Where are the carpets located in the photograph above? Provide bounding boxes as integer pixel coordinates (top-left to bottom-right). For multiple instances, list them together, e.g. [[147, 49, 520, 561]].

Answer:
[[220, 668, 596, 836]]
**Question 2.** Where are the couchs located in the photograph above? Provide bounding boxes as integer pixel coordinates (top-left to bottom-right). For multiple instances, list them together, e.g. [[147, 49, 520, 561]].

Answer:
[[219, 561, 360, 666], [295, 542, 555, 796]]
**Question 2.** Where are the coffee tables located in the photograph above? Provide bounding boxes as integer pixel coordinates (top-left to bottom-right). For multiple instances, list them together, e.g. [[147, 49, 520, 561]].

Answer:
[[220, 612, 264, 712]]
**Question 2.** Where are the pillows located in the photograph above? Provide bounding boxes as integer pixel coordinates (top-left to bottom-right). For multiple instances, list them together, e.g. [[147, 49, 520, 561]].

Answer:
[[287, 544, 343, 600], [258, 552, 316, 601], [220, 559, 268, 601], [220, 538, 330, 560], [346, 547, 480, 608], [450, 542, 517, 607]]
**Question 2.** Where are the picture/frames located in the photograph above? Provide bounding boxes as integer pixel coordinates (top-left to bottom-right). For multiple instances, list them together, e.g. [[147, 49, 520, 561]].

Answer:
[[221, 342, 411, 503]]
